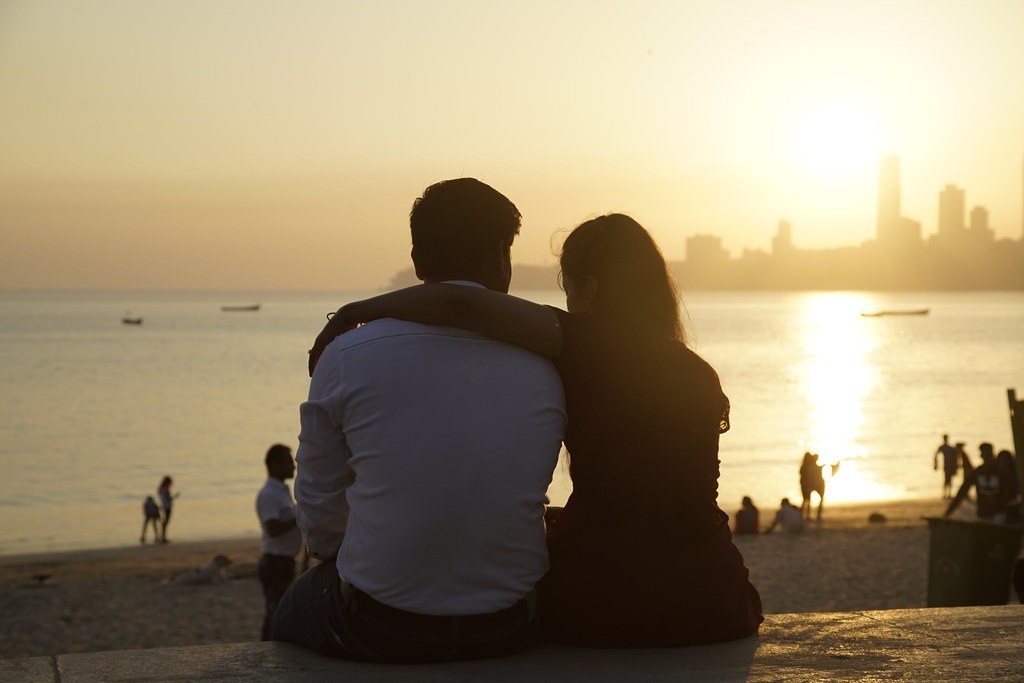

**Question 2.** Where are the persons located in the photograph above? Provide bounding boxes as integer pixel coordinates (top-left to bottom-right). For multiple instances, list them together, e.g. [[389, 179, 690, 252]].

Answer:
[[157, 476, 181, 544], [953, 443, 973, 499], [798, 452, 812, 522], [943, 442, 1003, 522], [933, 434, 958, 500], [139, 496, 161, 543], [762, 496, 804, 534], [806, 454, 825, 522], [271, 178, 572, 661], [993, 450, 1023, 525], [307, 212, 765, 652], [255, 443, 306, 642], [732, 495, 760, 535]]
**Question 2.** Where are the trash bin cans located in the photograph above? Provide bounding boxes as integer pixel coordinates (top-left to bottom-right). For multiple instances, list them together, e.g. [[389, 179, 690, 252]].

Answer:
[[921, 515, 1018, 607]]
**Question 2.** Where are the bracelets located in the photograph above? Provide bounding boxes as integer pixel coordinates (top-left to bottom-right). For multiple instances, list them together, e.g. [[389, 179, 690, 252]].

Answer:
[[326, 312, 362, 329]]
